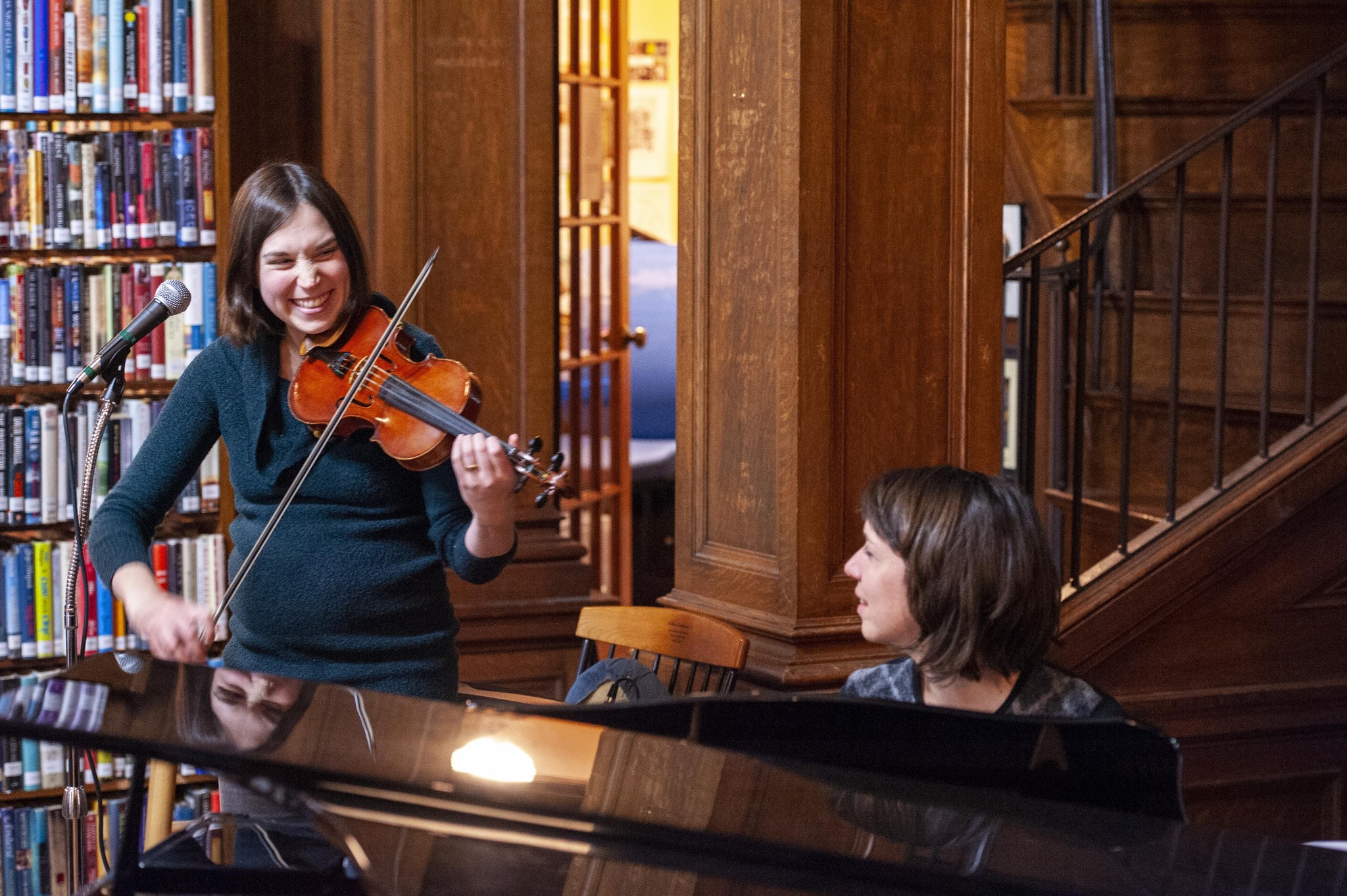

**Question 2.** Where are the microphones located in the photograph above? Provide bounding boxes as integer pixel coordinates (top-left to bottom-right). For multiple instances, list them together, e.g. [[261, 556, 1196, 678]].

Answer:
[[66, 279, 192, 396], [112, 650, 145, 673]]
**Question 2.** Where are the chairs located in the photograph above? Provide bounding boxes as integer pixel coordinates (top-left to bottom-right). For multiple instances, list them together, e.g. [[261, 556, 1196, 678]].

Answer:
[[575, 605, 751, 704]]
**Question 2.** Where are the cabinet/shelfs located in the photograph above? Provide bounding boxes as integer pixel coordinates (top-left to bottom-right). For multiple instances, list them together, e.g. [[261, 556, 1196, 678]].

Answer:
[[1, 0, 227, 896]]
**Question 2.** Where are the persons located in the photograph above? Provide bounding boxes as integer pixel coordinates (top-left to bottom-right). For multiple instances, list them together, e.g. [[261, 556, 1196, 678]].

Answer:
[[842, 466, 1123, 718], [173, 659, 314, 752], [88, 163, 527, 705]]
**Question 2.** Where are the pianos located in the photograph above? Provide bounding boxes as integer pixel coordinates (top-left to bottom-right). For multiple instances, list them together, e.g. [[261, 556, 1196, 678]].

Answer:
[[0, 647, 1347, 896]]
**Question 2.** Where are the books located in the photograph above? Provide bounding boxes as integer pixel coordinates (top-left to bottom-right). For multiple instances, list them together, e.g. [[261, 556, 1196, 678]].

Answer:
[[0, 0, 228, 896]]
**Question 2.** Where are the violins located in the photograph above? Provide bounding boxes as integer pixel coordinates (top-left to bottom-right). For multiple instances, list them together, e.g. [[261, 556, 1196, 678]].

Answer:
[[286, 303, 584, 513]]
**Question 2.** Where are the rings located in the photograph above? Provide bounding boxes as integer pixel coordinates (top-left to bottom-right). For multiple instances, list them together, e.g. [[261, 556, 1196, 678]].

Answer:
[[466, 464, 477, 471]]
